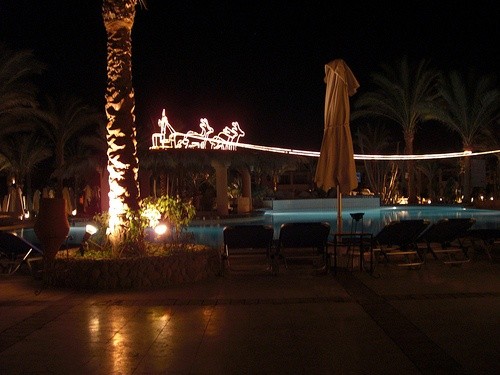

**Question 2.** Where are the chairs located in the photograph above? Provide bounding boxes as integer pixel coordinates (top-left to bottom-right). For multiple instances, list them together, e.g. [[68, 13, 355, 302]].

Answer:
[[220, 224, 276, 275], [0, 230, 45, 279], [271, 220, 331, 272], [366, 218, 500, 265]]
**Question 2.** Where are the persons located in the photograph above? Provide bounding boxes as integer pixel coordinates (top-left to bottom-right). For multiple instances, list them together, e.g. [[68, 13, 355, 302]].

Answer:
[[82, 240, 88, 248]]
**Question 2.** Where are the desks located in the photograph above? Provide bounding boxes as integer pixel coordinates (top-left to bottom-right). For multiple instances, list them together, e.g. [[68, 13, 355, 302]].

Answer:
[[332, 231, 377, 276]]
